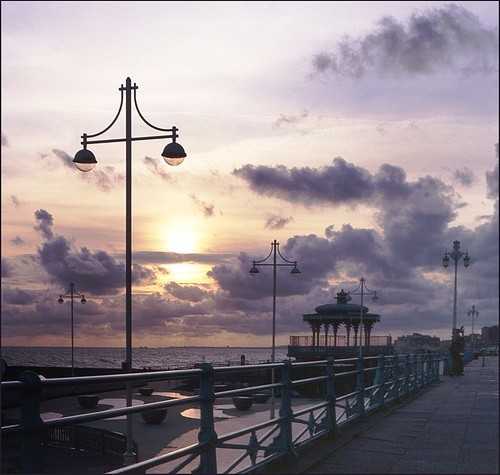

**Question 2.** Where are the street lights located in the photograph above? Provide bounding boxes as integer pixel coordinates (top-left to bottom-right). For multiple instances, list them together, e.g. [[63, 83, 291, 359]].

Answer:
[[441, 239, 471, 355], [72, 77, 189, 465], [58, 282, 87, 377], [467, 305, 480, 352], [345, 277, 379, 356], [248, 239, 301, 421]]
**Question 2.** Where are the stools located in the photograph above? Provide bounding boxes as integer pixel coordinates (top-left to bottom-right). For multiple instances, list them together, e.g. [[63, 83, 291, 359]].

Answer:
[[214, 385, 228, 392], [79, 395, 99, 407], [141, 410, 167, 423], [234, 397, 253, 409], [140, 388, 153, 395], [253, 394, 270, 403]]
